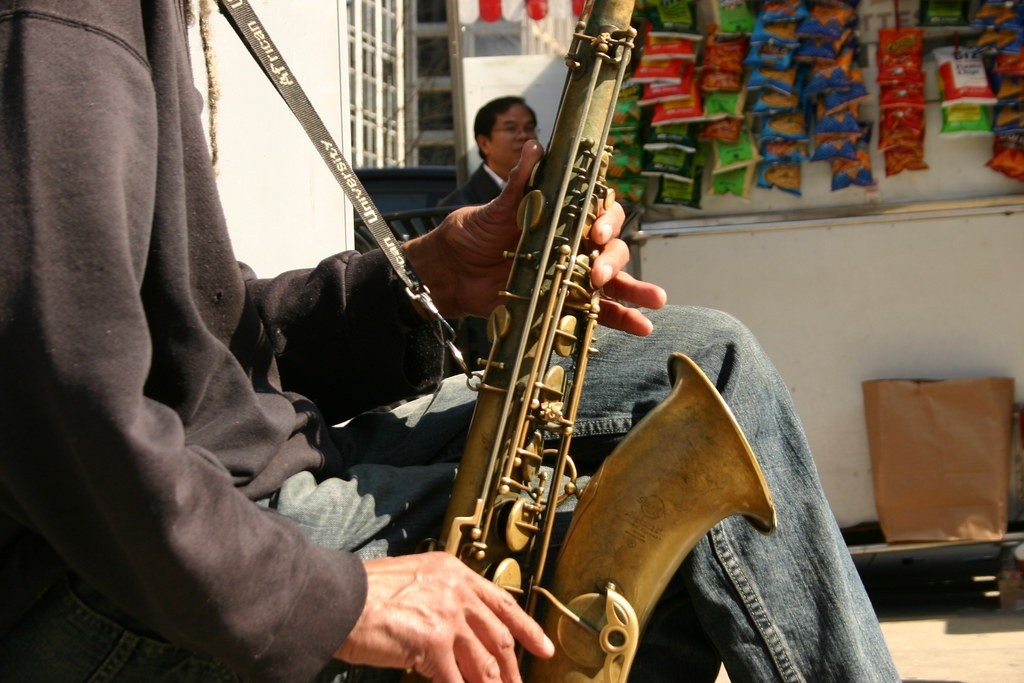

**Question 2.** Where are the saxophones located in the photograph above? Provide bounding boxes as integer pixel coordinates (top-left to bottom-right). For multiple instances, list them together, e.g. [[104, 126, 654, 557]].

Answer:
[[428, 0, 782, 682]]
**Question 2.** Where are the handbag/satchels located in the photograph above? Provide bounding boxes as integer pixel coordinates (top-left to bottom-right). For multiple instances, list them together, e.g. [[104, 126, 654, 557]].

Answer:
[[863, 377, 1016, 543]]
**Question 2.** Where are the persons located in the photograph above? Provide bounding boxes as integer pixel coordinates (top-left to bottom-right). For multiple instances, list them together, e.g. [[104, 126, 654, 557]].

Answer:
[[434, 96, 538, 223], [0, 0, 902, 683]]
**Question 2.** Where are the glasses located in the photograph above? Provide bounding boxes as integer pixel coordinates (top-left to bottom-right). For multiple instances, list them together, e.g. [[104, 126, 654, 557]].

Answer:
[[491, 120, 540, 137]]
